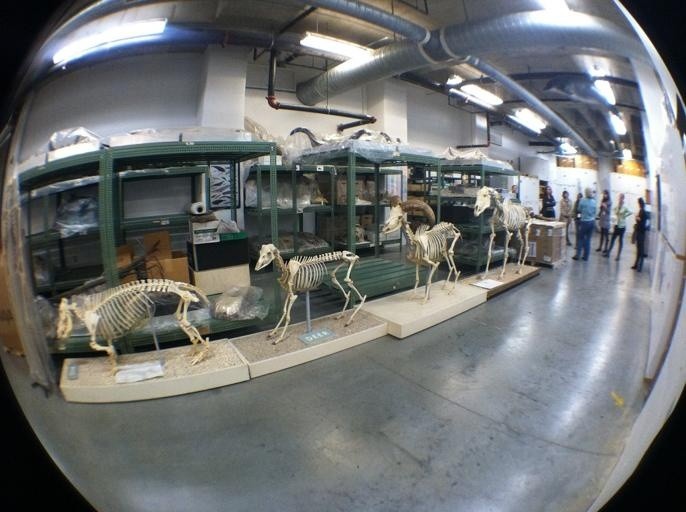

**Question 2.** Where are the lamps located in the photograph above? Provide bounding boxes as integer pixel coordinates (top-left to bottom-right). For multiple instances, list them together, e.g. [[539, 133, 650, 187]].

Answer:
[[299, 8, 376, 62]]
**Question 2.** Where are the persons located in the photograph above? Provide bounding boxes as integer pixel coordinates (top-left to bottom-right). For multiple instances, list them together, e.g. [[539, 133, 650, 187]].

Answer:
[[510, 185, 519, 199], [541, 186, 556, 219], [560, 191, 573, 248], [572, 188, 596, 262], [632, 197, 648, 272], [573, 192, 583, 250], [597, 190, 609, 253], [603, 194, 633, 262]]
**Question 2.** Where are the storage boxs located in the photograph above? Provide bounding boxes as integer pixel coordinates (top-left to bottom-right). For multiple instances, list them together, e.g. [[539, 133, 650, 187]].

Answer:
[[519, 219, 567, 265]]
